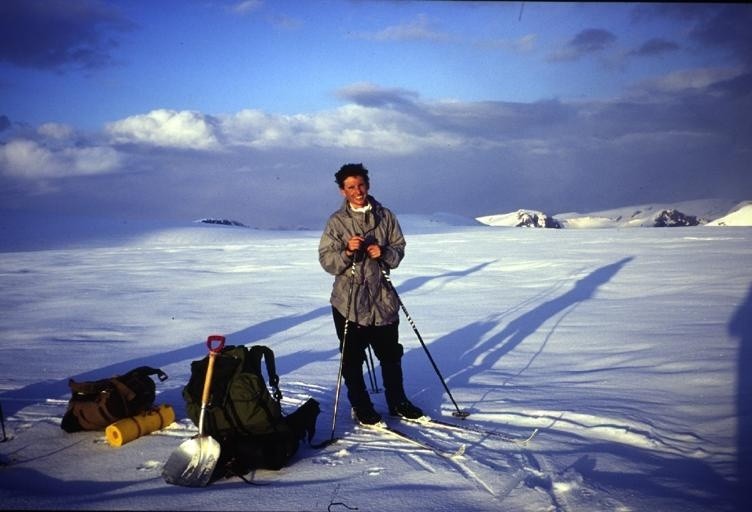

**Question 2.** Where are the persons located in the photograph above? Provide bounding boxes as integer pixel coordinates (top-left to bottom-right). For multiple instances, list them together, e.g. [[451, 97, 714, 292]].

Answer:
[[318, 162, 424, 425]]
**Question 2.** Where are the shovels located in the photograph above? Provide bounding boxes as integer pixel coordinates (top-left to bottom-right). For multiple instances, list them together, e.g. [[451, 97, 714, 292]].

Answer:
[[161, 335, 226, 488]]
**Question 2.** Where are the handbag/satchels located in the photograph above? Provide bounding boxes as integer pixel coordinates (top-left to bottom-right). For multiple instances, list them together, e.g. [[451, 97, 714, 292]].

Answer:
[[60, 364, 170, 435]]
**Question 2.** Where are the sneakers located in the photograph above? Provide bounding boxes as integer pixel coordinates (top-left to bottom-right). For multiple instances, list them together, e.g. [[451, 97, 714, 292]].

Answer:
[[387, 393, 423, 420], [351, 399, 382, 425]]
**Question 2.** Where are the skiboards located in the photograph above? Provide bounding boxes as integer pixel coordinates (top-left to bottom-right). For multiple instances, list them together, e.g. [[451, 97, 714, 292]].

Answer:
[[353, 403, 539, 458]]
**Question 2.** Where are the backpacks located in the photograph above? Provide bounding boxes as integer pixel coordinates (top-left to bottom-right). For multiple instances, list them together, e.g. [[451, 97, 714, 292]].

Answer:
[[180, 343, 283, 436]]
